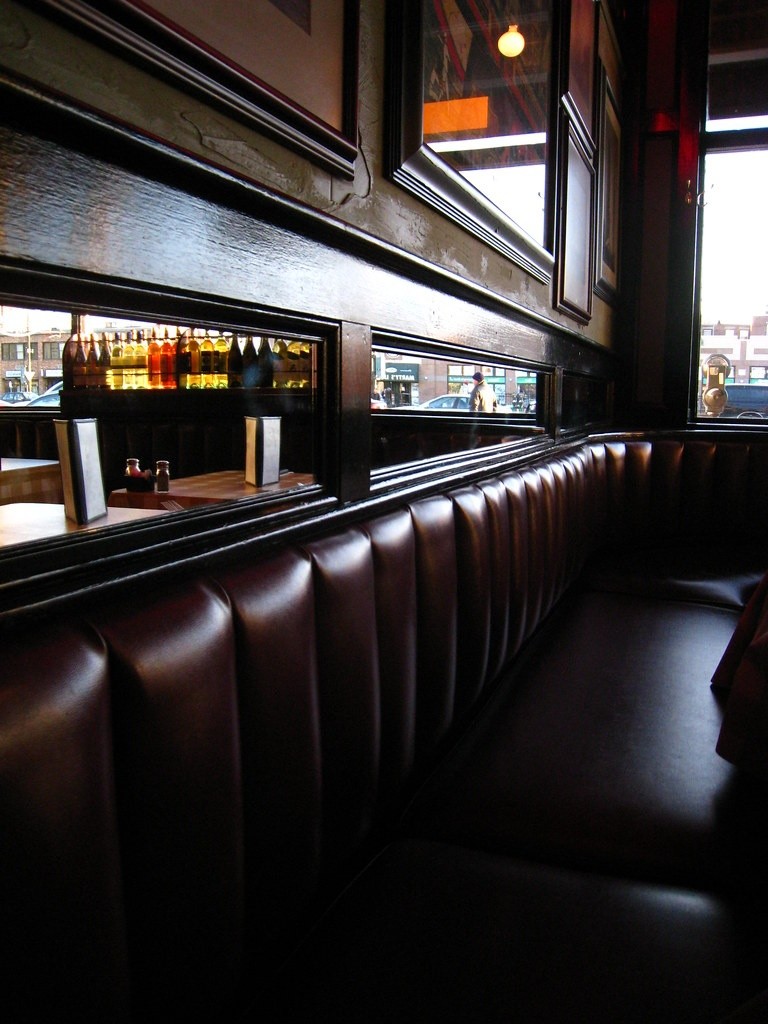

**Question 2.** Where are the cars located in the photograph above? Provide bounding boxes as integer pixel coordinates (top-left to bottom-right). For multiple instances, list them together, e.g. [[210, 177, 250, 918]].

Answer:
[[396, 393, 474, 410], [0, 380, 65, 406]]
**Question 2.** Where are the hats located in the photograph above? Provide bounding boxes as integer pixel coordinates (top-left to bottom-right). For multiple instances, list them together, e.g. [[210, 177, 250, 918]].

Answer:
[[472, 372, 484, 383]]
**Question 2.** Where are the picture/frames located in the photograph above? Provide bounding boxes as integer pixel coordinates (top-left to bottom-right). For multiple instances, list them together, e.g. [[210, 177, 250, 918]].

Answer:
[[551, 114, 597, 326], [592, 55, 626, 310], [380, 0, 573, 285], [13, 0, 360, 180]]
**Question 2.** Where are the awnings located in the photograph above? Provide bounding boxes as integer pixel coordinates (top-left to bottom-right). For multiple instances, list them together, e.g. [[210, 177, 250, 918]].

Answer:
[[382, 364, 419, 383]]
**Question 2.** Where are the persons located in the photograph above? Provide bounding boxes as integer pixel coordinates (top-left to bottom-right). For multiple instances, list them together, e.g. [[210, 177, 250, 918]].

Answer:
[[469, 372, 498, 413], [371, 388, 380, 400], [459, 380, 470, 394]]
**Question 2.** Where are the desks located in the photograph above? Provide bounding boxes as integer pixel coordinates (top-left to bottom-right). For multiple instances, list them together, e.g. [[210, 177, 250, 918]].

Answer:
[[107, 471, 315, 512], [0, 502, 171, 548], [0, 458, 64, 505]]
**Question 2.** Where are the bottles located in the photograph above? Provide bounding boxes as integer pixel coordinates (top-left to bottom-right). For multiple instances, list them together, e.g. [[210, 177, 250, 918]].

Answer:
[[257, 337, 273, 387], [99, 332, 111, 388], [300, 342, 310, 388], [228, 336, 243, 388], [273, 339, 288, 388], [126, 459, 141, 475], [156, 461, 170, 491], [73, 337, 86, 389], [84, 337, 89, 356], [173, 329, 187, 387], [134, 331, 148, 388], [87, 333, 100, 388], [200, 333, 214, 388], [242, 335, 257, 387], [214, 335, 228, 388], [148, 329, 160, 388], [287, 341, 300, 387], [161, 329, 176, 389], [188, 332, 200, 387], [122, 331, 135, 386], [112, 333, 123, 388]]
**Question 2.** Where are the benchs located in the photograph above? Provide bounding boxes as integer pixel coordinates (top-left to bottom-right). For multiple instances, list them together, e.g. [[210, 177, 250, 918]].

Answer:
[[0, 427, 768, 1024]]
